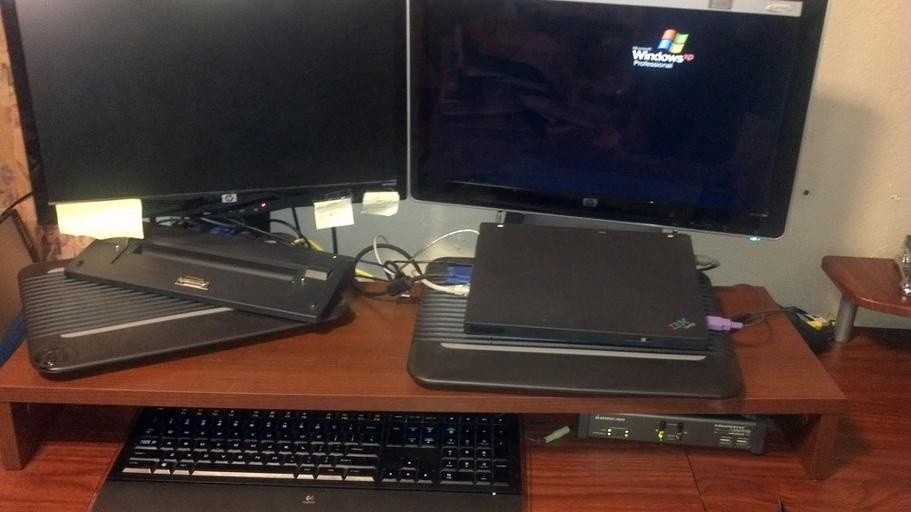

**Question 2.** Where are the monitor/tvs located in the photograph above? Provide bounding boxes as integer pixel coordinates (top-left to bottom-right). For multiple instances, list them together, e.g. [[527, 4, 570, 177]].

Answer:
[[408, 0, 831, 239], [0, 0, 409, 227]]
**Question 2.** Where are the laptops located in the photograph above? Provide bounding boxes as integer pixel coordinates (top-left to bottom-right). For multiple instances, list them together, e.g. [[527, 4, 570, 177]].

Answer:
[[463, 222, 709, 351]]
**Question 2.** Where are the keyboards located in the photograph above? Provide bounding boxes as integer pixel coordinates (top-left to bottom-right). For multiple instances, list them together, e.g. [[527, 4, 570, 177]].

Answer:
[[62, 235, 357, 324], [91, 406, 522, 512]]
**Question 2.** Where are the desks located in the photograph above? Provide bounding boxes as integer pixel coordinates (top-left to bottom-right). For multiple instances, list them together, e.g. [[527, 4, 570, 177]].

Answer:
[[0, 253, 910, 512]]
